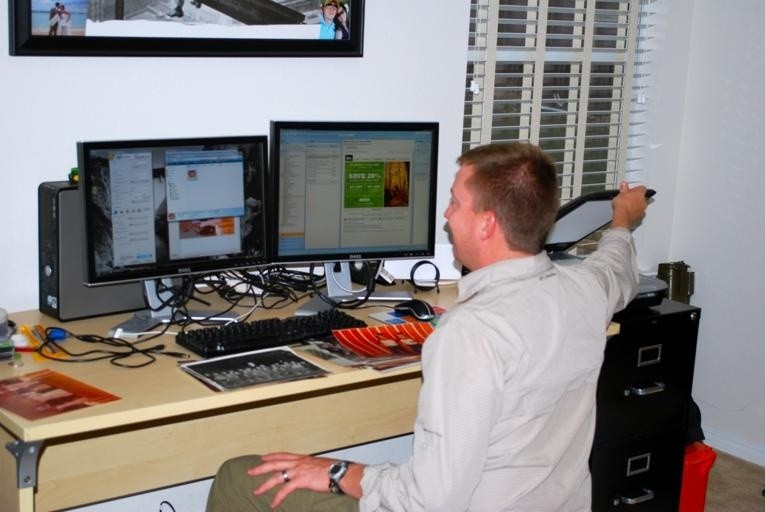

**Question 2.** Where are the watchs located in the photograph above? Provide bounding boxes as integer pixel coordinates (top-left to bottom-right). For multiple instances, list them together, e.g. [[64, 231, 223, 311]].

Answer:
[[326, 458, 356, 497]]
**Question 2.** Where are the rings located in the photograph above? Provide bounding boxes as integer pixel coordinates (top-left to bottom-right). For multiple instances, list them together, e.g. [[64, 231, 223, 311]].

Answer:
[[282, 467, 291, 482]]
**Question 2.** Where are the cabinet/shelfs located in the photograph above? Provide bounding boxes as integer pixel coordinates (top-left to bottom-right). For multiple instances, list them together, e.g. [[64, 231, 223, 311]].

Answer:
[[589, 300, 700, 512]]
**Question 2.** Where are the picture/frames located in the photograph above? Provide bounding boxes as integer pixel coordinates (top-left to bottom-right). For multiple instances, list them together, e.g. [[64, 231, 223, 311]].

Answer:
[[6, 0, 365, 60]]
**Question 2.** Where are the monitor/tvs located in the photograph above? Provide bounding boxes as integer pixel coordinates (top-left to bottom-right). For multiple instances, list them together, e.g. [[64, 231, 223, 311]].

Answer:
[[269, 121, 440, 317], [77, 134, 270, 337]]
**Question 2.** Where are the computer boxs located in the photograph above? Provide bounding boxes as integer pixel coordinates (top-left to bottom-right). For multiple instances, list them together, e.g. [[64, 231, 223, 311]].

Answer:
[[38, 182, 195, 322]]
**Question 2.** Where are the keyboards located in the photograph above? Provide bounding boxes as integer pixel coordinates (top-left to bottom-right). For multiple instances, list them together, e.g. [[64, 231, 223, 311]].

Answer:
[[176, 309, 368, 359]]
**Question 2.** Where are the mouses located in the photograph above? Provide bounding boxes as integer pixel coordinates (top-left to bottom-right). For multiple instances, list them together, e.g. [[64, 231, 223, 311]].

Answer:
[[394, 299, 435, 321]]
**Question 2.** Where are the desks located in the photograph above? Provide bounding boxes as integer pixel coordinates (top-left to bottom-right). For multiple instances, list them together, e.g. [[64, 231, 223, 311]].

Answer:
[[0, 283, 620, 512]]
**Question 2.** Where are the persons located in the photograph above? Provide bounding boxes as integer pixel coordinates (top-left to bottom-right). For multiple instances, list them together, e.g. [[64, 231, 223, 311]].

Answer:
[[333, 3, 351, 40], [376, 332, 406, 356], [318, 0, 338, 40], [394, 329, 423, 355], [46, 2, 70, 37], [204, 140, 651, 511], [57, 4, 70, 37]]
